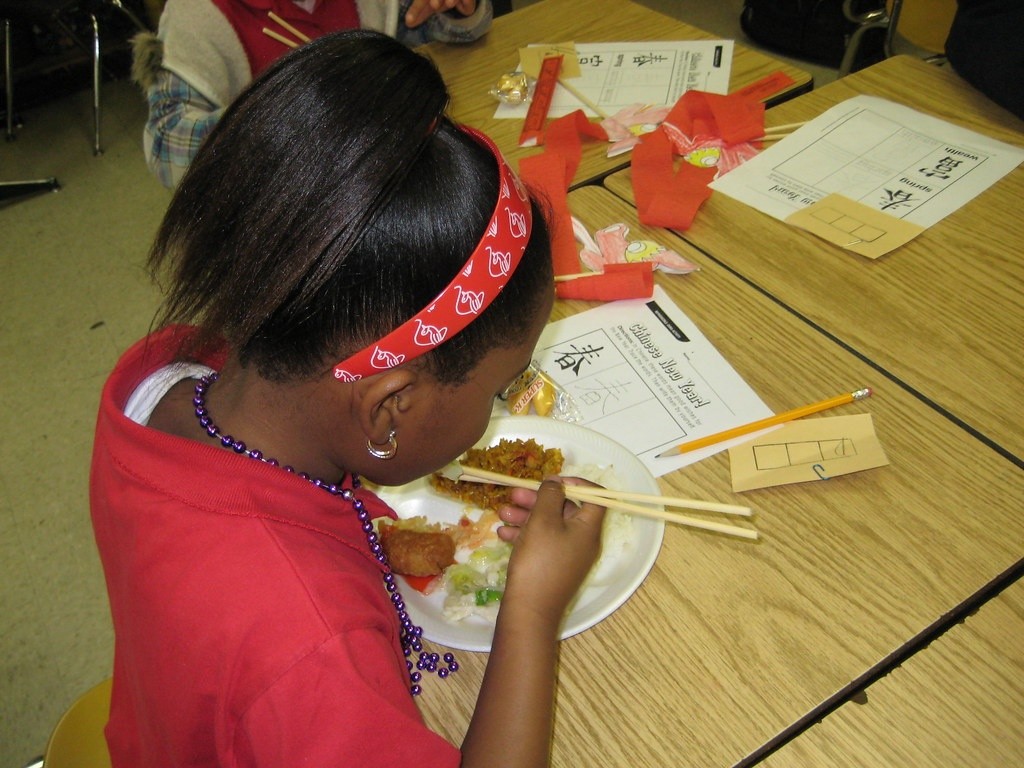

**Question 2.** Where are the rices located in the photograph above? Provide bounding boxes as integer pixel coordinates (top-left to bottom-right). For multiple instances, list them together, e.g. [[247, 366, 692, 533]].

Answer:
[[559, 463, 640, 568]]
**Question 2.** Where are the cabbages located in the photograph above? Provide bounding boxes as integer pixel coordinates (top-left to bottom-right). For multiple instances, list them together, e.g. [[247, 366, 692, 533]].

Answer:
[[441, 544, 512, 622]]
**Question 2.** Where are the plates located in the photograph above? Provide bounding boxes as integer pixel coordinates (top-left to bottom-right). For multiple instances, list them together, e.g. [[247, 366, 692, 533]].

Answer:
[[357, 416, 666, 653]]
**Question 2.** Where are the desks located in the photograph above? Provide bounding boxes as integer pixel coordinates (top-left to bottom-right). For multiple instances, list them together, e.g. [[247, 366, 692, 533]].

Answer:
[[605, 55, 1024, 468], [412, 0, 813, 193], [751, 574, 1024, 768], [398, 184, 1024, 768]]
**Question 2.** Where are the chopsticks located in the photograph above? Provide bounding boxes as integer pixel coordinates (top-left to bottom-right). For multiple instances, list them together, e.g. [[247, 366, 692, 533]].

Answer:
[[262, 12, 312, 48], [437, 467, 757, 540], [751, 122, 806, 141]]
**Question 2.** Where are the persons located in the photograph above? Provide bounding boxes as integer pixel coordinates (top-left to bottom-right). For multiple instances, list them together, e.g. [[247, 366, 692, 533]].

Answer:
[[141, 1, 495, 189], [89, 27, 607, 768]]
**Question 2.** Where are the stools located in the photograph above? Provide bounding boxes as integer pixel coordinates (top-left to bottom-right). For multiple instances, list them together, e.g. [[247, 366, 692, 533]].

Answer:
[[24, 679, 112, 768]]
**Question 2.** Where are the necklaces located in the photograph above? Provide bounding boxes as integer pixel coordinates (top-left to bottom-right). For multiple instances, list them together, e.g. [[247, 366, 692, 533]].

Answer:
[[191, 374, 461, 700]]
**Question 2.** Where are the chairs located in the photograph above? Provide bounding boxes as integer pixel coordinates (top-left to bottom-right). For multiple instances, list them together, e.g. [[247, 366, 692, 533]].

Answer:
[[838, 0, 960, 72], [0, 0, 152, 156]]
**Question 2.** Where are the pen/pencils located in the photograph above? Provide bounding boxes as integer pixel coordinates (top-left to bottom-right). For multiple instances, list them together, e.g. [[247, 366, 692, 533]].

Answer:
[[654, 386, 873, 460]]
